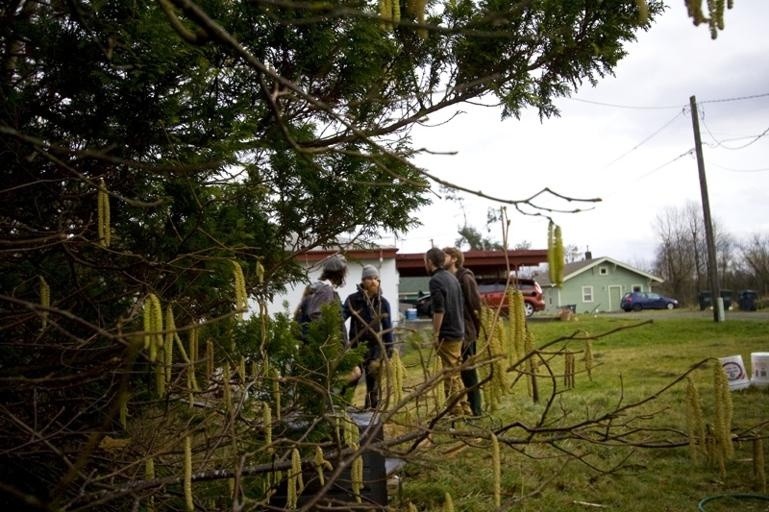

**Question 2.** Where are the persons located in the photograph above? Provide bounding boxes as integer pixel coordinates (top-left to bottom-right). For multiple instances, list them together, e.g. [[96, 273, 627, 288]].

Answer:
[[294, 253, 347, 343], [341, 264, 393, 410], [424, 248, 474, 417], [442, 248, 482, 417]]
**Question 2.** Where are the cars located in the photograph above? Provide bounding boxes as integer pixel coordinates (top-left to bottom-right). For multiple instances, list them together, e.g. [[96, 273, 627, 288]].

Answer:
[[620, 292, 680, 312]]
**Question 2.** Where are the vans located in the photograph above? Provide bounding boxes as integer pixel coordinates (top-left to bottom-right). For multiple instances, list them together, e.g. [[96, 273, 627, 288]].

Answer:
[[418, 277, 547, 322]]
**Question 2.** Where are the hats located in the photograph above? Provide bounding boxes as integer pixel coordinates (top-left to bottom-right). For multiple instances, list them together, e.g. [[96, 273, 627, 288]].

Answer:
[[322, 254, 347, 272], [363, 264, 380, 280]]
